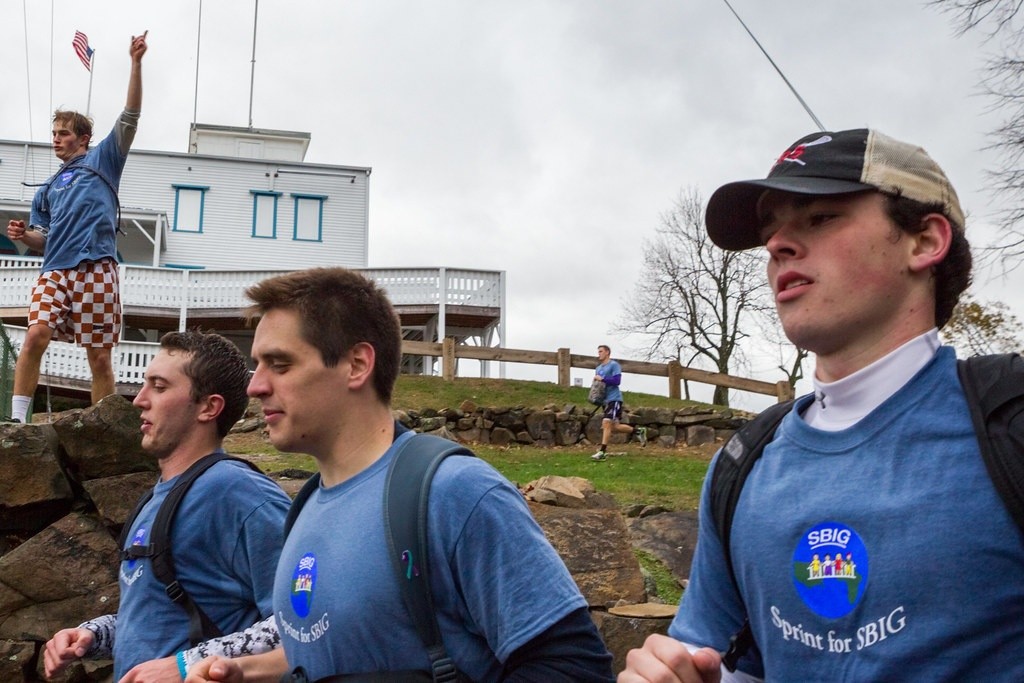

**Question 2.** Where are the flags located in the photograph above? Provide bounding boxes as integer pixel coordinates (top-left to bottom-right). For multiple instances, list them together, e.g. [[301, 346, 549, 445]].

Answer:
[[72, 29, 94, 72]]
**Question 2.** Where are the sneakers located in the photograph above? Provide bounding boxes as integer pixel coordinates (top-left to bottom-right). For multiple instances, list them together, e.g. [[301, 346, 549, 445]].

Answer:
[[636, 428, 647, 449], [590, 451, 606, 462]]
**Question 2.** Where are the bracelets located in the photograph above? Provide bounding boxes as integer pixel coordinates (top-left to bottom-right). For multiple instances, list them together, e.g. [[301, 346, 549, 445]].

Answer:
[[176, 652, 187, 680]]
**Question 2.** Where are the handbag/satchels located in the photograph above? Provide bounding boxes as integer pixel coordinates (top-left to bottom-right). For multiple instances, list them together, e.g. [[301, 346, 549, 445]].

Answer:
[[588, 381, 606, 406]]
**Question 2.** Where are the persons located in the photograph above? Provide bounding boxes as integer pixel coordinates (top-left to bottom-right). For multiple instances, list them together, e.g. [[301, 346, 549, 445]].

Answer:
[[0, 28, 148, 424], [590, 345, 647, 462], [617, 128, 1024, 683], [42, 330, 297, 683], [184, 268, 615, 683]]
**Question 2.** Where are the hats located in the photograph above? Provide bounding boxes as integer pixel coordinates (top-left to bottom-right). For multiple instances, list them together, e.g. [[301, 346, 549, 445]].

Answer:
[[705, 128, 965, 251]]
